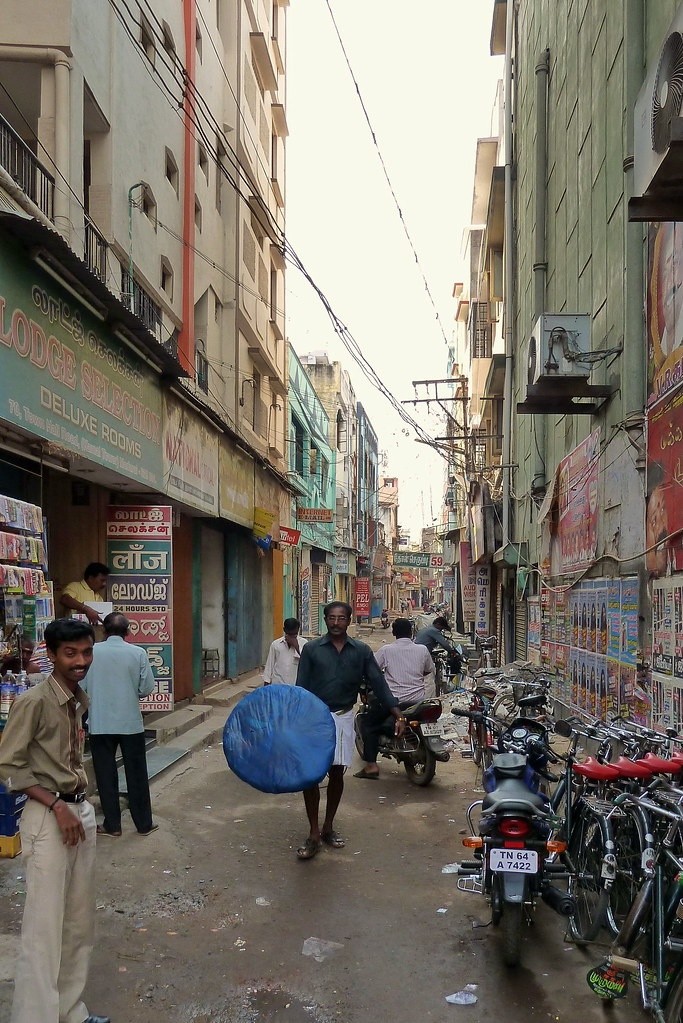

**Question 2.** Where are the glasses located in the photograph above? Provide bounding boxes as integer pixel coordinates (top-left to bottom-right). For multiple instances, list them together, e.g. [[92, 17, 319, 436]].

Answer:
[[326, 616, 348, 621]]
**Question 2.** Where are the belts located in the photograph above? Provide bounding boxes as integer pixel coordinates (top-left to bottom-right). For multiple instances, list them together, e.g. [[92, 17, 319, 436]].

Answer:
[[30, 792, 87, 804]]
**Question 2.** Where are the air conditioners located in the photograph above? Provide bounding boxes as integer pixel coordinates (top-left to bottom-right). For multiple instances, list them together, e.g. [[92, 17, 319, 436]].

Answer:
[[523, 313, 591, 403], [451, 502, 457, 510], [447, 492, 453, 498], [446, 497, 455, 506], [633, 0, 683, 195]]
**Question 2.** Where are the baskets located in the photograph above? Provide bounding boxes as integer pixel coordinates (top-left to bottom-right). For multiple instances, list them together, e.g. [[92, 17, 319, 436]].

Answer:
[[513, 676, 546, 707]]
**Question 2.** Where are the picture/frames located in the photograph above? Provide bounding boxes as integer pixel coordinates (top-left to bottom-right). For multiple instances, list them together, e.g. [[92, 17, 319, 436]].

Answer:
[[71, 479, 90, 505]]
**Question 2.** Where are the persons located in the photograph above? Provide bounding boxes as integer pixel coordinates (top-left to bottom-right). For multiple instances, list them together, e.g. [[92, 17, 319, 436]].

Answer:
[[0, 618, 111, 1023], [660, 222, 683, 354], [59, 563, 111, 640], [77, 613, 160, 836], [398, 597, 415, 616], [572, 659, 606, 698], [296, 601, 407, 857], [353, 617, 435, 777], [414, 616, 450, 697], [647, 461, 668, 574], [380, 608, 388, 621], [562, 525, 596, 563], [264, 617, 309, 688], [573, 602, 607, 631]]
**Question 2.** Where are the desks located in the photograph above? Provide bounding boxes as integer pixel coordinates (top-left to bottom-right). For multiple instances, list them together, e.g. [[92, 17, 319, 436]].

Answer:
[[357, 628, 371, 637], [466, 650, 498, 675], [360, 624, 375, 633]]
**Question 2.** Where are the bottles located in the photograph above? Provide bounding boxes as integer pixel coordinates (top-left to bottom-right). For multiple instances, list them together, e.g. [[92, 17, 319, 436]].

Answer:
[[0, 670, 18, 720], [16, 670, 31, 695]]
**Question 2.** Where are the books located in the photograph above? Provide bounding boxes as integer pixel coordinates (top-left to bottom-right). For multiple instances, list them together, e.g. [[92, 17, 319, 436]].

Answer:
[[0, 496, 55, 642]]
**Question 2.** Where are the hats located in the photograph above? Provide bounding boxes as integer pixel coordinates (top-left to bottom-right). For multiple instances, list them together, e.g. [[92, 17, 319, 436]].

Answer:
[[437, 617, 451, 631]]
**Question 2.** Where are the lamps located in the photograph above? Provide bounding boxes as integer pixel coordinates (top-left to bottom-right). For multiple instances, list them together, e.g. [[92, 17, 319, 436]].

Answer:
[[0, 436, 70, 473], [30, 245, 109, 322], [232, 439, 261, 465], [166, 380, 229, 436], [110, 320, 169, 379]]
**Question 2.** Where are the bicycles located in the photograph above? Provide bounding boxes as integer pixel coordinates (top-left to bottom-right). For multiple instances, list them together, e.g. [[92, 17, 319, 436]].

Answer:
[[544, 710, 683, 1023], [467, 631, 499, 668], [431, 641, 449, 697], [444, 671, 504, 785], [492, 661, 556, 745]]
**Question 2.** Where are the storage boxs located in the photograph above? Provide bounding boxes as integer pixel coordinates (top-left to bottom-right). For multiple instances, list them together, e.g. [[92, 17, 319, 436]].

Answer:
[[0, 781, 30, 858]]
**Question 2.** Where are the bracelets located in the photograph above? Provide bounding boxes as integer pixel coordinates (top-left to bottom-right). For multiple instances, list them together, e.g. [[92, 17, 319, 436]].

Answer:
[[50, 797, 61, 811], [396, 718, 407, 722]]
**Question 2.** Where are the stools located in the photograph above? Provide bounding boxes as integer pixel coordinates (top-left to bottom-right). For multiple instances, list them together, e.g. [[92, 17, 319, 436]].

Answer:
[[201, 648, 222, 678]]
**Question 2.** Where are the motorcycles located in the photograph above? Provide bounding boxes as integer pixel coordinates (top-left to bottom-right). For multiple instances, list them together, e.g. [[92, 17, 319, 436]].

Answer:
[[381, 609, 390, 629], [450, 707, 575, 968], [355, 672, 450, 786]]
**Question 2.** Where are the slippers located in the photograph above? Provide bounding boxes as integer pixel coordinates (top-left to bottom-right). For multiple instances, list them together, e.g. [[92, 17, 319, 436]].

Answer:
[[297, 839, 322, 858], [353, 768, 379, 778], [96, 824, 119, 838], [321, 831, 345, 848], [138, 823, 159, 835]]
